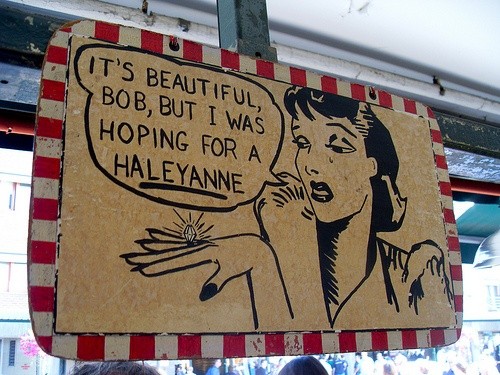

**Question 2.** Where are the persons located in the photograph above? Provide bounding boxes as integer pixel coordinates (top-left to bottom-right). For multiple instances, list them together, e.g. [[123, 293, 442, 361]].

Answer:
[[70, 331, 500, 375]]
